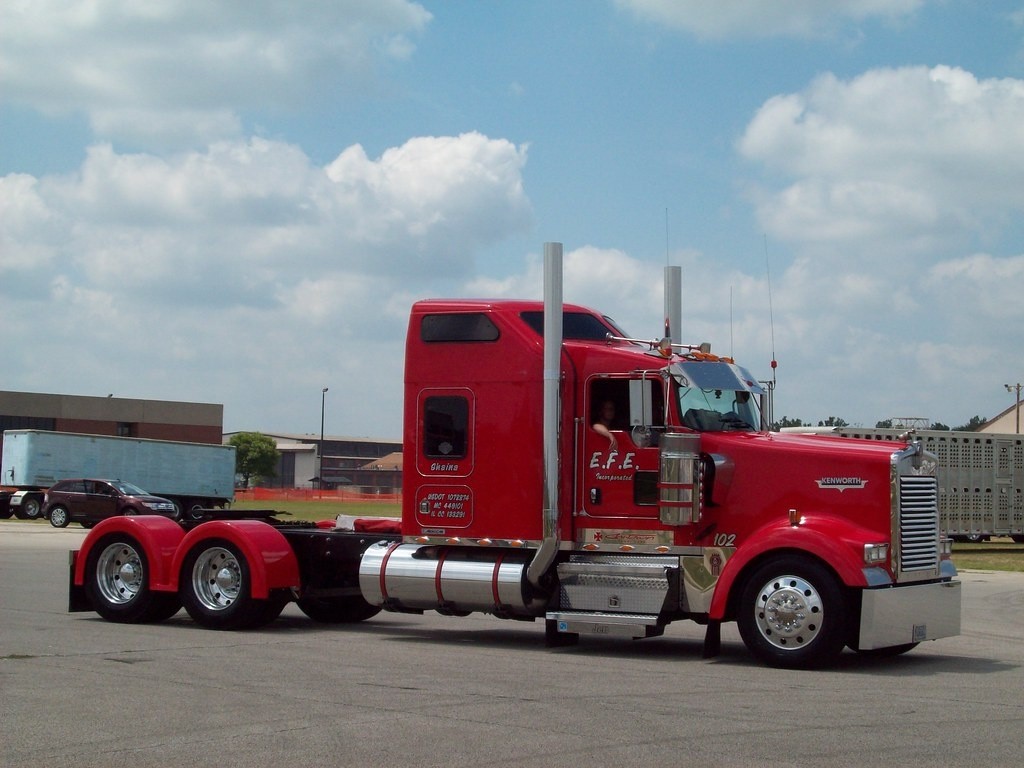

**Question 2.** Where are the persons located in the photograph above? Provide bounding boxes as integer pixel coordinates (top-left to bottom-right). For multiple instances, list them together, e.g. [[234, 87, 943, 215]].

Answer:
[[593, 400, 619, 451]]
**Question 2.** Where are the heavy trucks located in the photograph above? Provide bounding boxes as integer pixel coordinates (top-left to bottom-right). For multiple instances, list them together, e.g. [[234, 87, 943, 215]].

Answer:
[[63, 241, 962, 669]]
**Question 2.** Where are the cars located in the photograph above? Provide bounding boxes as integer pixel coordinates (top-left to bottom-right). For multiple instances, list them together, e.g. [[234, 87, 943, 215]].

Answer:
[[40, 477, 180, 530]]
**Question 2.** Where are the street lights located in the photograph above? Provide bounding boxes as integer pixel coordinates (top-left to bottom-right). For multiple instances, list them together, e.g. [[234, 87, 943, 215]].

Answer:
[[318, 387, 329, 500]]
